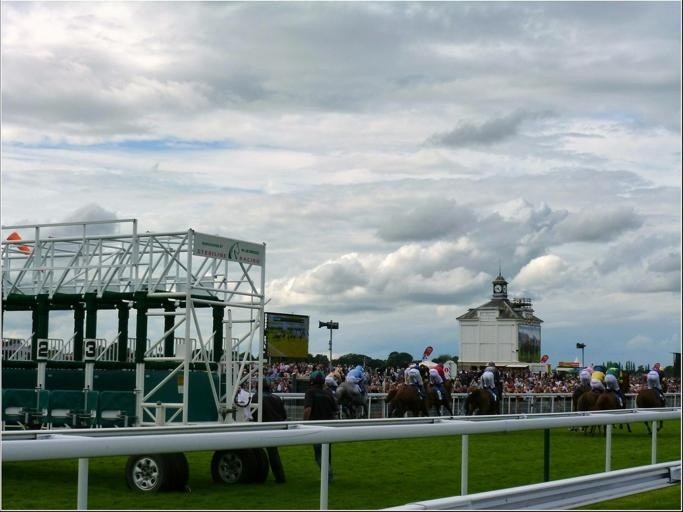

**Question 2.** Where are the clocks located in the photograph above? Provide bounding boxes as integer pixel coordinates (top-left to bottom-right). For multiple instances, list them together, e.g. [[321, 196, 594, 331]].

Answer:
[[494, 285, 502, 293], [503, 285, 507, 293]]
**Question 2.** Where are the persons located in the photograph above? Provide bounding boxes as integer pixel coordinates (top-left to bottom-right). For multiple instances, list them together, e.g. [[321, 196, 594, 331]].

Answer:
[[251, 379, 287, 484], [304, 370, 338, 478], [243, 361, 681, 407]]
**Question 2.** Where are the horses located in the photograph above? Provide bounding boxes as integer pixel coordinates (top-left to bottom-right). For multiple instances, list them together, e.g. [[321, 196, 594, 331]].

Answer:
[[384, 381, 455, 423], [462, 381, 503, 416], [636, 387, 666, 437], [570, 381, 633, 437], [336, 380, 369, 419]]
